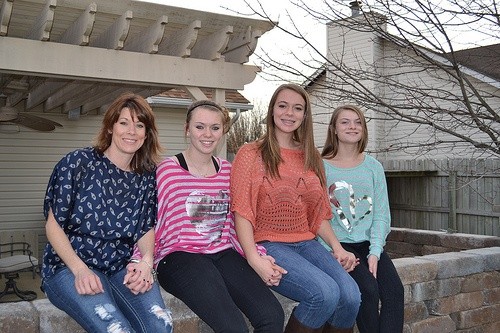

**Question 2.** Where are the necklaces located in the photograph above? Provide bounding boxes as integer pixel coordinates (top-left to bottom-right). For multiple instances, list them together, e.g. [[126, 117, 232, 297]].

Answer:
[[188, 155, 212, 177]]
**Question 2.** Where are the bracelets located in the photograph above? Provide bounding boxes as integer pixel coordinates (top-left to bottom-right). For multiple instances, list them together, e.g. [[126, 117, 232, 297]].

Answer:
[[141, 259, 158, 282], [127, 258, 140, 263]]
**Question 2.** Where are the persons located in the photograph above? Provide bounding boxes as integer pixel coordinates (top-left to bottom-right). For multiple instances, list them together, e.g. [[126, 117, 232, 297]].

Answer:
[[229, 84, 362, 333], [316, 105, 405, 333], [41, 93, 174, 333], [123, 99, 285, 333]]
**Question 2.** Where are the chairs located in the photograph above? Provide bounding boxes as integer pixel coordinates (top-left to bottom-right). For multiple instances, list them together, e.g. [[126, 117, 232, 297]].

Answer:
[[0, 242, 39, 303]]
